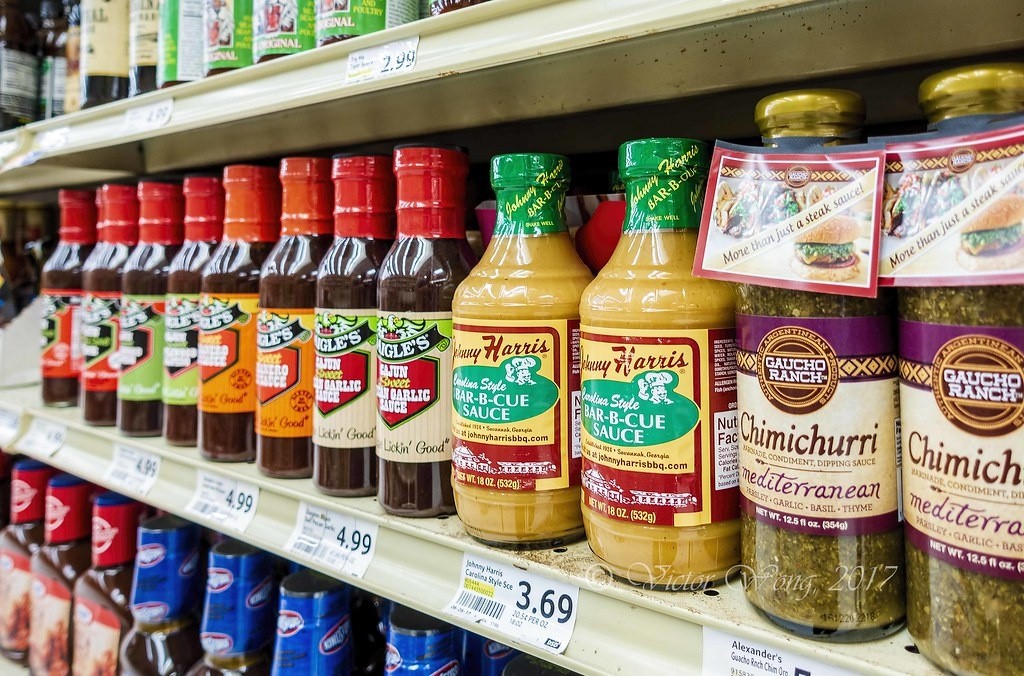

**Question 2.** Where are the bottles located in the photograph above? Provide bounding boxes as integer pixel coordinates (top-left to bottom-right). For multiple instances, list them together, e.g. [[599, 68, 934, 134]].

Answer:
[[0, 0, 1024, 676]]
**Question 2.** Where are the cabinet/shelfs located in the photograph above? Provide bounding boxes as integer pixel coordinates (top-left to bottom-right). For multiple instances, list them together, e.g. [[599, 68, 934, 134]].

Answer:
[[0, 1, 1024, 676]]
